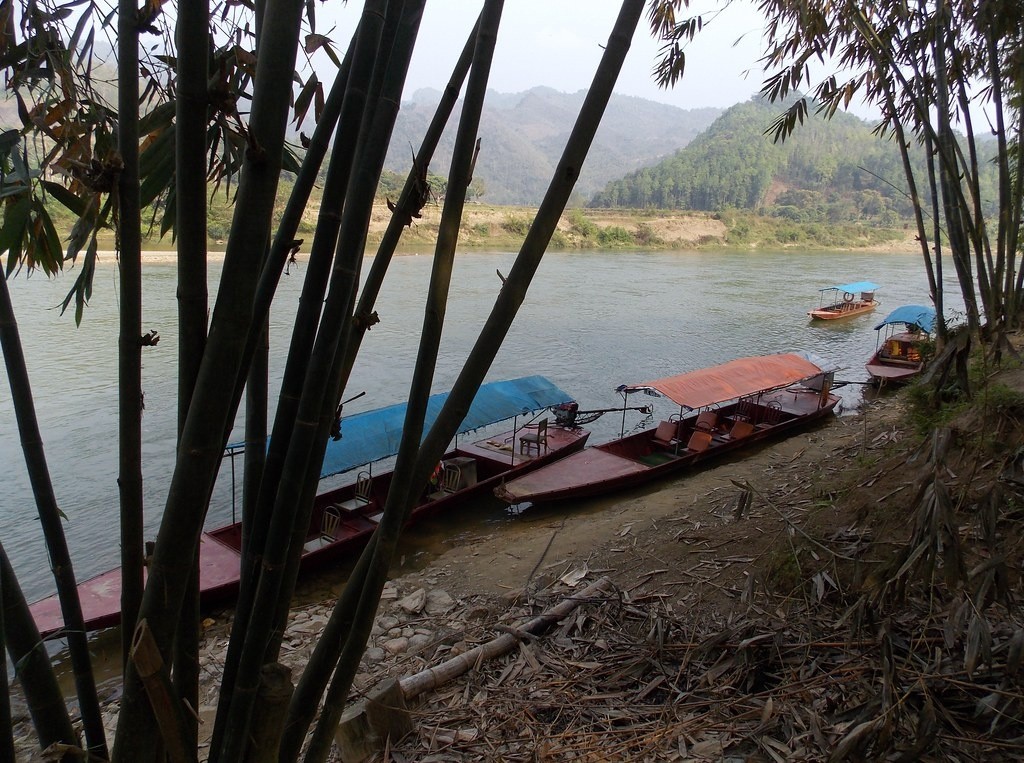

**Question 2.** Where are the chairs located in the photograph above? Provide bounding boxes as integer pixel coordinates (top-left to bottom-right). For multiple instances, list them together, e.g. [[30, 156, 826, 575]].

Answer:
[[650, 393, 782, 455], [426, 462, 461, 502], [519, 418, 549, 458], [334, 470, 373, 521], [302, 505, 341, 552]]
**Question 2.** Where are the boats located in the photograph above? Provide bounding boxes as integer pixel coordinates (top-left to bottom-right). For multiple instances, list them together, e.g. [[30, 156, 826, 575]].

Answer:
[[864, 304, 937, 381], [807, 281, 881, 320], [29, 374, 591, 641], [493, 350, 843, 504]]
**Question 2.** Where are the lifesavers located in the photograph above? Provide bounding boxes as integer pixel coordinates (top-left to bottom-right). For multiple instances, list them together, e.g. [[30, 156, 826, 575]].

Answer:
[[843, 291, 854, 302]]
